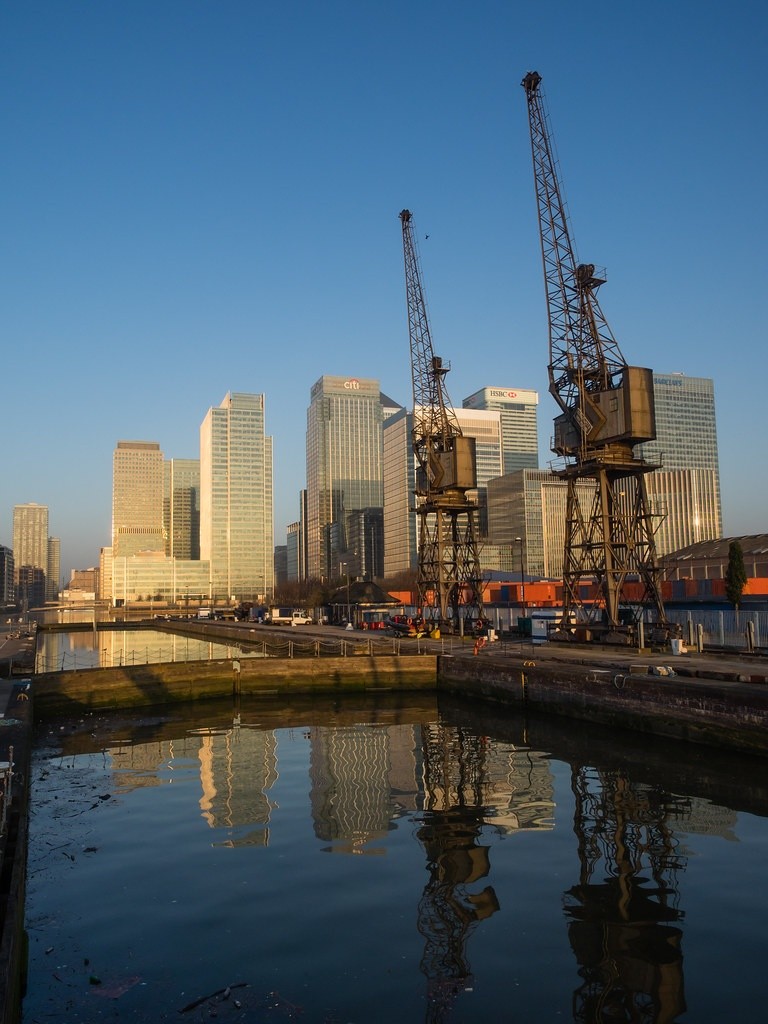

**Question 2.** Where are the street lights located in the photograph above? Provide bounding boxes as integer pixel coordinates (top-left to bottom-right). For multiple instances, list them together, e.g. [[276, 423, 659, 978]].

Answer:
[[343, 562, 354, 631], [515, 536, 526, 617]]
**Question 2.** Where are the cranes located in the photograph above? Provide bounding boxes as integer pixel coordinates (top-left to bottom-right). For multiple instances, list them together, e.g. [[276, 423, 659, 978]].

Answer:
[[396, 205, 491, 637], [518, 69, 684, 655]]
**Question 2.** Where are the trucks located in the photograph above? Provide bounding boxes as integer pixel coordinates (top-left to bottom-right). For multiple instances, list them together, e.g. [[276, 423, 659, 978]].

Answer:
[[270, 611, 312, 626]]
